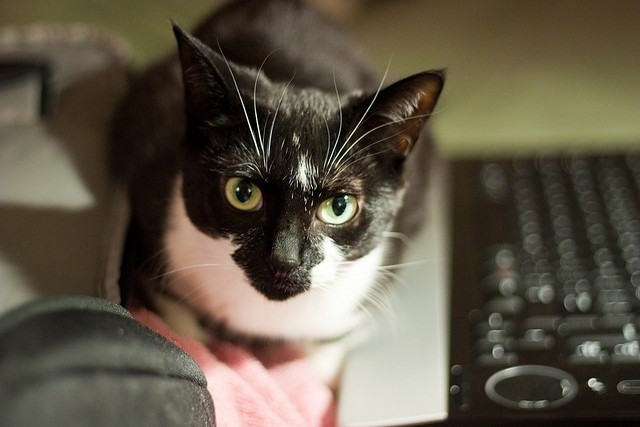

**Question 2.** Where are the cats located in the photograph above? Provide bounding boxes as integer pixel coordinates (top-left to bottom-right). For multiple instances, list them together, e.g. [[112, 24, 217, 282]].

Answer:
[[106, 0, 448, 345]]
